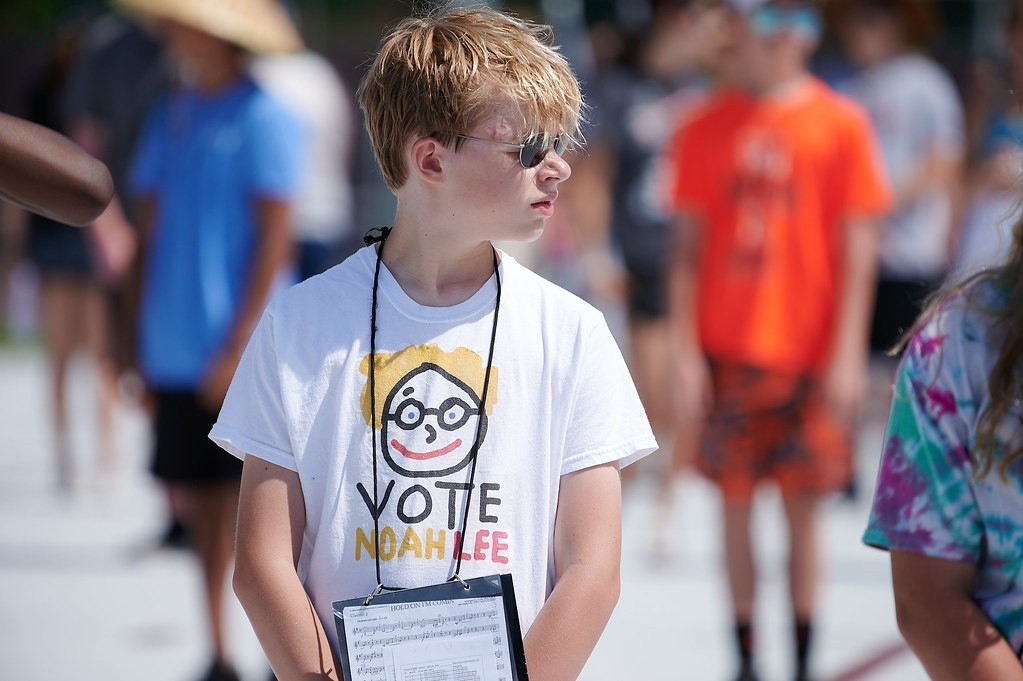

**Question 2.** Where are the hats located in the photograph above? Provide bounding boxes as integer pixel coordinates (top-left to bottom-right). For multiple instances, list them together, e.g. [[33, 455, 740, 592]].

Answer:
[[122, 0, 303, 55]]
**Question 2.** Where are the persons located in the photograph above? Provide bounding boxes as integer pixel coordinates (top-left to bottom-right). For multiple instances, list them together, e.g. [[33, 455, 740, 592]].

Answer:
[[208, 7, 659, 681], [667, 3, 899, 681], [2, 4, 1021, 552], [862, 185, 1023, 680]]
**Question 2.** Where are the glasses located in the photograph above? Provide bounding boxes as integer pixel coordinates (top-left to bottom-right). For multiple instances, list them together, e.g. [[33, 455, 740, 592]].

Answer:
[[428, 128, 570, 170]]
